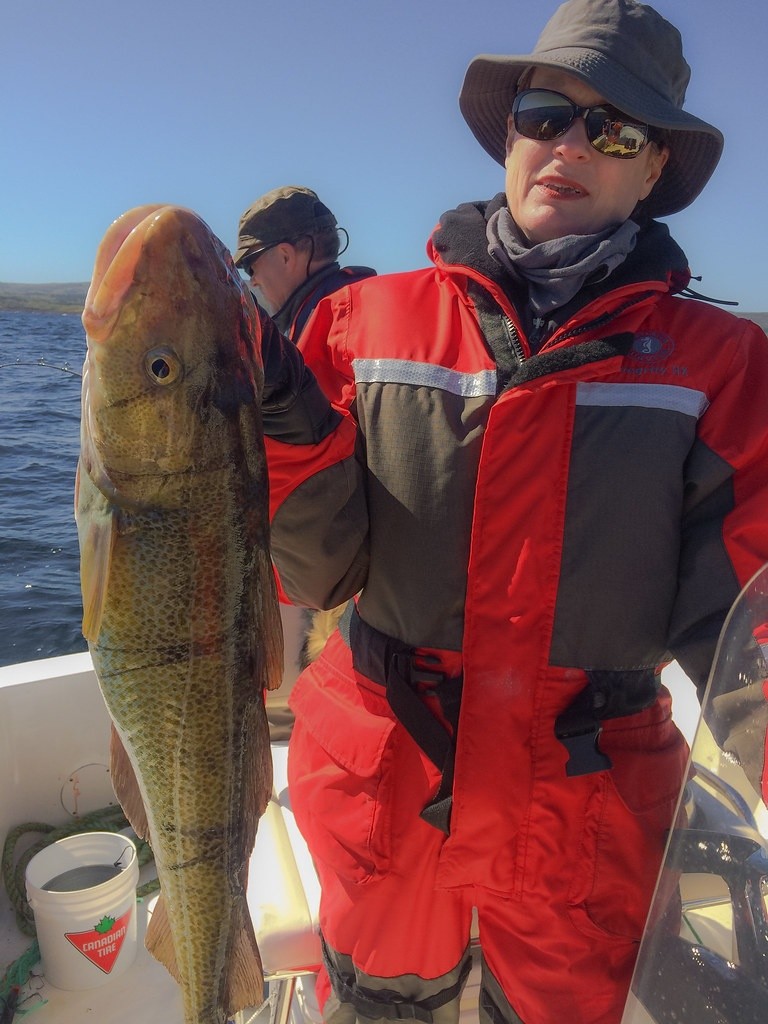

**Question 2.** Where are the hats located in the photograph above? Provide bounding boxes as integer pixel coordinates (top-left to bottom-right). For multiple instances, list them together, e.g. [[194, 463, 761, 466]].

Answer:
[[460, 1, 724, 217], [231, 186, 337, 269]]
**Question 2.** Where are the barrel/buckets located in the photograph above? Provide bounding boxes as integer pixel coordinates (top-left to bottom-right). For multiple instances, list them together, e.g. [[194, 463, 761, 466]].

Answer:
[[25, 832, 140, 992]]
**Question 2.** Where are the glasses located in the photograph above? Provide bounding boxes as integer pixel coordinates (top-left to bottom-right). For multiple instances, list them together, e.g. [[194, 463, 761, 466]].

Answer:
[[509, 88, 658, 160], [239, 238, 299, 277]]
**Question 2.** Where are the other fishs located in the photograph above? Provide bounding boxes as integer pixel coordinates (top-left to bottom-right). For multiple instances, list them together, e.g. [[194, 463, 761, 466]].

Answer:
[[74, 205, 287, 1024]]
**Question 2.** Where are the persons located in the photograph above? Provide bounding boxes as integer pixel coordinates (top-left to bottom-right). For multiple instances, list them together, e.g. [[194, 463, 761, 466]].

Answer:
[[259, 0, 768, 1024], [234, 185, 377, 347]]
